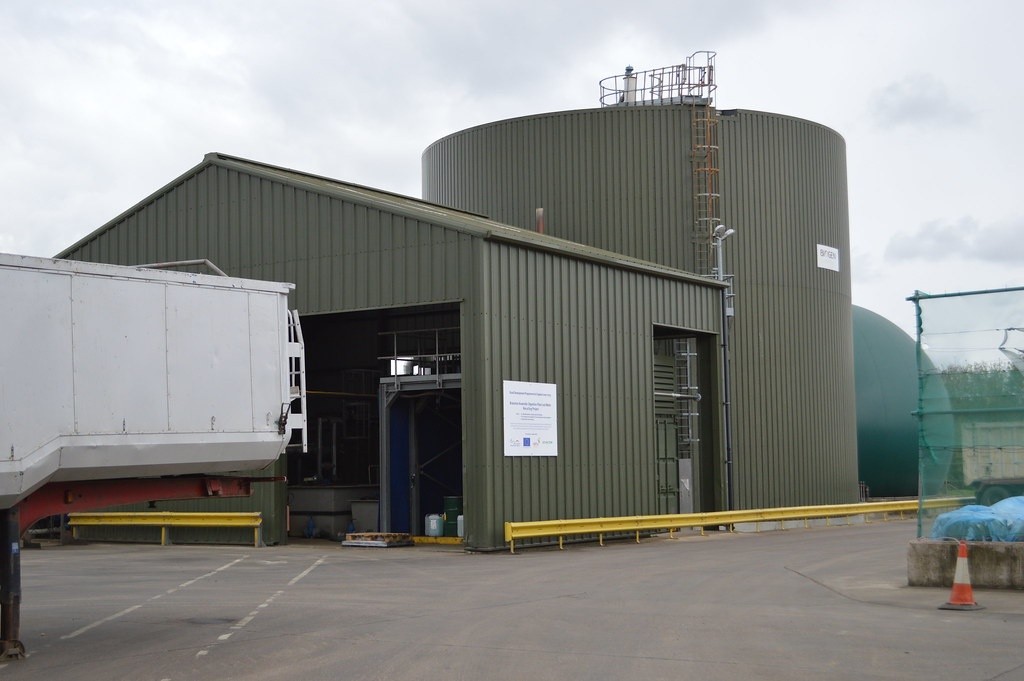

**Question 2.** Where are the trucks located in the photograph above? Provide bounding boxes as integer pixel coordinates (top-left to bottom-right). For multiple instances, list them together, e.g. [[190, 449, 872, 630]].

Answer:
[[961, 420, 1024, 508]]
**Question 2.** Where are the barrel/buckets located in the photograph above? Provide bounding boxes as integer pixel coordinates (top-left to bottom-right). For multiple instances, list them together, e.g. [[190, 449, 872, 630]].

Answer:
[[443, 496, 463, 537], [425, 514, 444, 536], [457, 515, 463, 536]]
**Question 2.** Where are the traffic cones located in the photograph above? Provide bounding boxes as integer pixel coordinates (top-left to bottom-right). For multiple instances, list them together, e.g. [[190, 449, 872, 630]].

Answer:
[[937, 540, 986, 611]]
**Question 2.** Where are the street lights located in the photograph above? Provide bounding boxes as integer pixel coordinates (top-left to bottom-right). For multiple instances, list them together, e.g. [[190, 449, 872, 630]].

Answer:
[[713, 224, 736, 279]]
